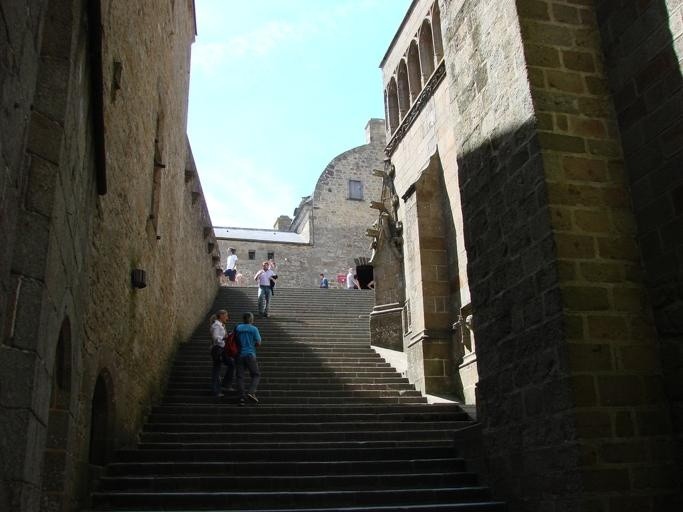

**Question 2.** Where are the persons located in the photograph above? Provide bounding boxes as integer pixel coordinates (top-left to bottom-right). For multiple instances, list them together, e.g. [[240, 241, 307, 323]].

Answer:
[[318, 274, 327, 288], [207, 308, 234, 398], [336, 266, 361, 290], [253, 262, 277, 318], [231, 312, 262, 405], [220, 246, 238, 287]]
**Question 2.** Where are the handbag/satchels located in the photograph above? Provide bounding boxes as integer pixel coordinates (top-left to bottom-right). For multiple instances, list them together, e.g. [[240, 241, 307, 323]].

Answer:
[[224, 333, 241, 358]]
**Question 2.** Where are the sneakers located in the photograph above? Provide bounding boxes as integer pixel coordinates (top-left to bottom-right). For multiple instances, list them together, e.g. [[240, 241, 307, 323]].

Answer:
[[219, 388, 258, 407]]
